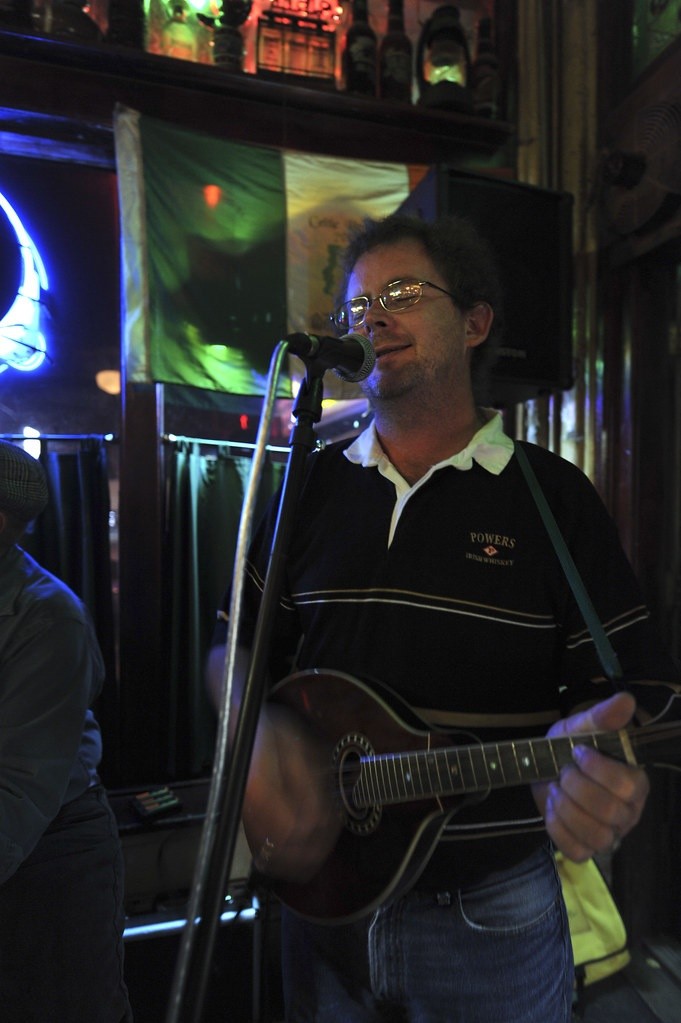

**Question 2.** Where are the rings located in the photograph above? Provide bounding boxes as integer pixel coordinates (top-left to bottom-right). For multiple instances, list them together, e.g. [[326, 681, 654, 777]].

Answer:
[[259, 836, 279, 864]]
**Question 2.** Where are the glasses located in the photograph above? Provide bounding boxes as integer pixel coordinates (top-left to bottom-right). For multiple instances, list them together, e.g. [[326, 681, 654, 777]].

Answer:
[[327, 277, 463, 329]]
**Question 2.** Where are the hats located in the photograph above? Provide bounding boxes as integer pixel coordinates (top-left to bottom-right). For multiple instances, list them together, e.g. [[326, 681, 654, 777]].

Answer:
[[0, 439, 49, 519]]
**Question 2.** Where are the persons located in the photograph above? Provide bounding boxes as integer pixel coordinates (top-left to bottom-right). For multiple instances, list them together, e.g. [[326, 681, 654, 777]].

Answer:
[[0, 437, 127, 1022], [209, 216, 651, 1023]]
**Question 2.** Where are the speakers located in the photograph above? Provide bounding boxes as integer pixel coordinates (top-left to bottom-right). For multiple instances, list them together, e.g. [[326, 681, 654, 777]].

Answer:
[[396, 166, 573, 411]]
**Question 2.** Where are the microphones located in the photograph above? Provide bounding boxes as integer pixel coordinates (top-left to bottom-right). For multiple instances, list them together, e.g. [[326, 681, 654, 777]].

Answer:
[[281, 333, 376, 382]]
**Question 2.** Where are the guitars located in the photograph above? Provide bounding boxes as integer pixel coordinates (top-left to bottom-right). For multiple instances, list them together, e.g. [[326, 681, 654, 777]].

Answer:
[[241, 667, 680, 928]]
